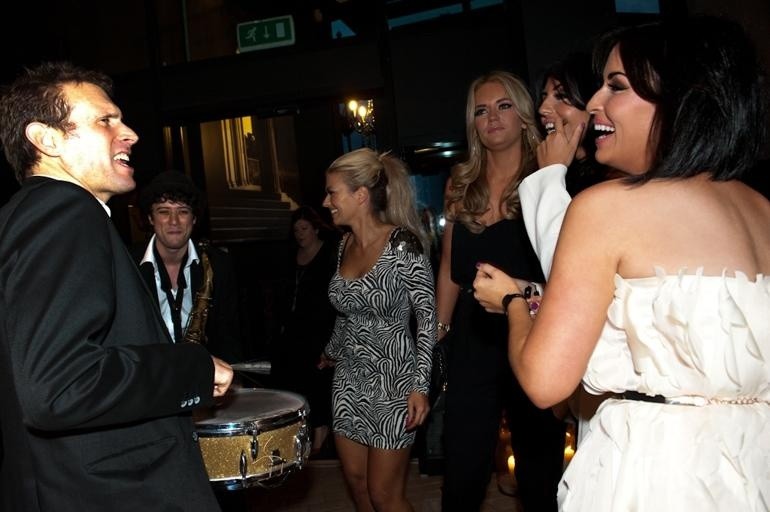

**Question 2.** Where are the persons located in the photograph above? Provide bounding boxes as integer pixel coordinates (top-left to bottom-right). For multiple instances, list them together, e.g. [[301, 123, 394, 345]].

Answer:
[[317, 148, 435, 510], [435, 71, 565, 509], [279, 204, 338, 454], [474, 18, 768, 510], [0, 57, 234, 509], [129, 180, 234, 364], [517, 59, 629, 420]]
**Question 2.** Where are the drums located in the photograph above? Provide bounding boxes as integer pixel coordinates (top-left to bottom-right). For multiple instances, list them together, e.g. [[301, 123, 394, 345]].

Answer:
[[197, 387, 314, 490]]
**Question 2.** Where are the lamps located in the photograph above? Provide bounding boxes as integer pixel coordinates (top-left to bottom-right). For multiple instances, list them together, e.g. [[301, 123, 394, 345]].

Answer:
[[346, 98, 378, 136]]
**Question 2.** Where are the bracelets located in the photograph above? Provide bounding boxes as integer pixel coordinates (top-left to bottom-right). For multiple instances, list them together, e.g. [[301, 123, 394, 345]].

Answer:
[[434, 320, 452, 334], [503, 292, 524, 312]]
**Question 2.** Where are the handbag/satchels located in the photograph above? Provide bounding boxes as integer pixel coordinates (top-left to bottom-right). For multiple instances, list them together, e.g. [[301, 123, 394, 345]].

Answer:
[[428, 327, 453, 413]]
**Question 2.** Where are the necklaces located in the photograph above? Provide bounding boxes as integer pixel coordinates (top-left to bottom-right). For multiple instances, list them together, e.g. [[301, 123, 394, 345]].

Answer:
[[295, 243, 318, 311]]
